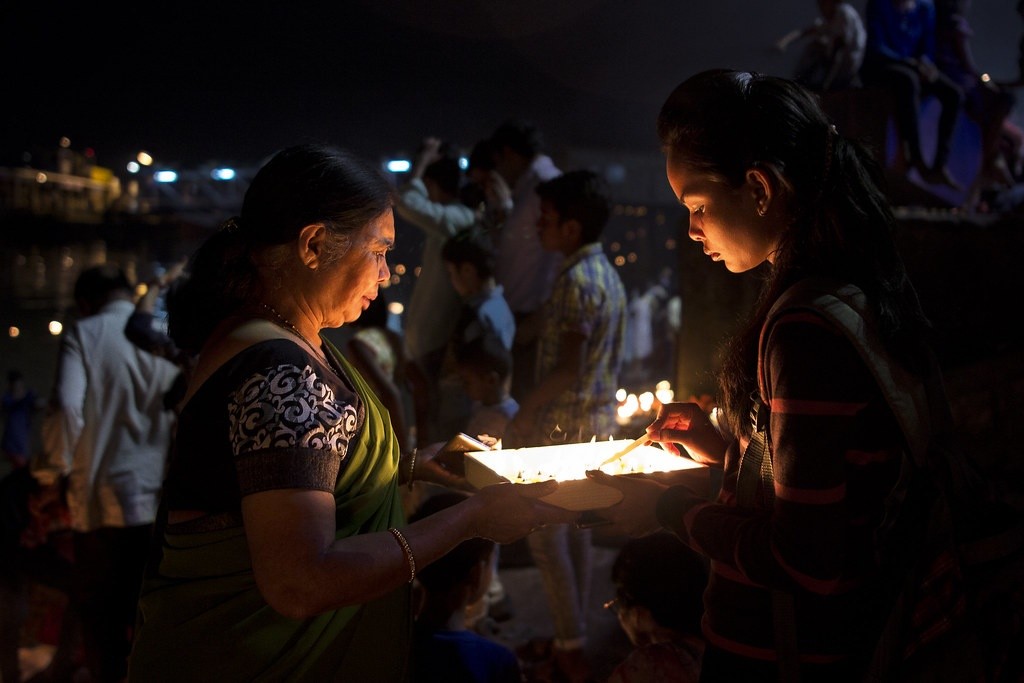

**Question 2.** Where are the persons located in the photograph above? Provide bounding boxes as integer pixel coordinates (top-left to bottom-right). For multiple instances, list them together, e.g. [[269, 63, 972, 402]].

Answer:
[[127, 142, 582, 683], [586, 68, 950, 683], [345, 121, 629, 683], [777, 0, 1024, 214], [0, 208, 192, 683]]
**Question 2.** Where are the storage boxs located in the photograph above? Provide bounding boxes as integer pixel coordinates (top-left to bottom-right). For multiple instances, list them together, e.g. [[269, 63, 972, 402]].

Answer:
[[463, 438, 710, 511]]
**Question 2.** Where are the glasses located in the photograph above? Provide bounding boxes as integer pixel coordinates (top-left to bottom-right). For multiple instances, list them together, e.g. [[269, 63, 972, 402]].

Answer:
[[602, 596, 620, 616]]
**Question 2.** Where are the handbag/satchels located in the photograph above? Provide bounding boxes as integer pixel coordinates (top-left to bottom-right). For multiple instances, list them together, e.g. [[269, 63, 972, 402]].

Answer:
[[701, 296, 1024, 683]]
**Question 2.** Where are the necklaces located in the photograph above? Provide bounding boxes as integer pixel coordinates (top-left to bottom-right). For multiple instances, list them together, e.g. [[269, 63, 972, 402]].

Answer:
[[255, 296, 339, 376]]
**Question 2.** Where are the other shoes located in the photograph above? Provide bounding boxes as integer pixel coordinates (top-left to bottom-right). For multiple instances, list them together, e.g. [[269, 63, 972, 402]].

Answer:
[[480, 587, 515, 625], [526, 635, 587, 659]]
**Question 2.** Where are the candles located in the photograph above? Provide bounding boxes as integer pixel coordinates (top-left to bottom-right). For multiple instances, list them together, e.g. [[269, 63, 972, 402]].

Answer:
[[598, 434, 649, 470]]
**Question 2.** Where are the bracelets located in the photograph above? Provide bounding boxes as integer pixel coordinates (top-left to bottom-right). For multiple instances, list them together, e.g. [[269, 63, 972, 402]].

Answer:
[[409, 448, 417, 489], [389, 528, 417, 583]]
